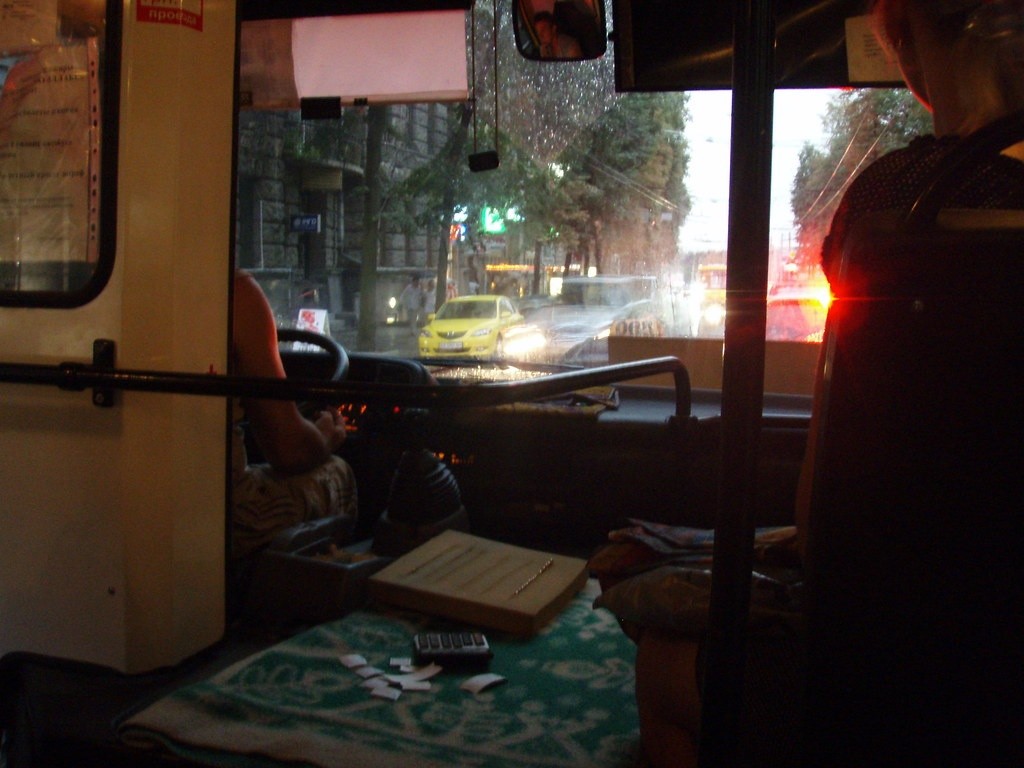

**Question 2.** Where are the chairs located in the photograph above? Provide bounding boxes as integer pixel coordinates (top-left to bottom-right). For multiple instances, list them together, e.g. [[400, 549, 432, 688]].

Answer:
[[804, 109, 1024, 768]]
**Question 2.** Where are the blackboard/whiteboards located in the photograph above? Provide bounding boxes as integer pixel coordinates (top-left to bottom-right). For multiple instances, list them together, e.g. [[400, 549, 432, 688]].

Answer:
[[293, 308, 326, 353]]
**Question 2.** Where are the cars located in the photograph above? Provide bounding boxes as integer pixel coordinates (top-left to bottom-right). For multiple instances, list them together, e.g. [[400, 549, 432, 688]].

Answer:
[[693, 262, 729, 306], [417, 296, 525, 360]]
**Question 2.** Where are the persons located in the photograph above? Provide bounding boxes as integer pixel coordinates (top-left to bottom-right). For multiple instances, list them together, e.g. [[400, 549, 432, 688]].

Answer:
[[535, 10, 582, 62], [821, 0, 1024, 299], [230, 270, 359, 535]]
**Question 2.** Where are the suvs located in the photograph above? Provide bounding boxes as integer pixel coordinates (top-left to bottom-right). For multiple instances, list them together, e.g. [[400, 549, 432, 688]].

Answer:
[[527, 274, 667, 357]]
